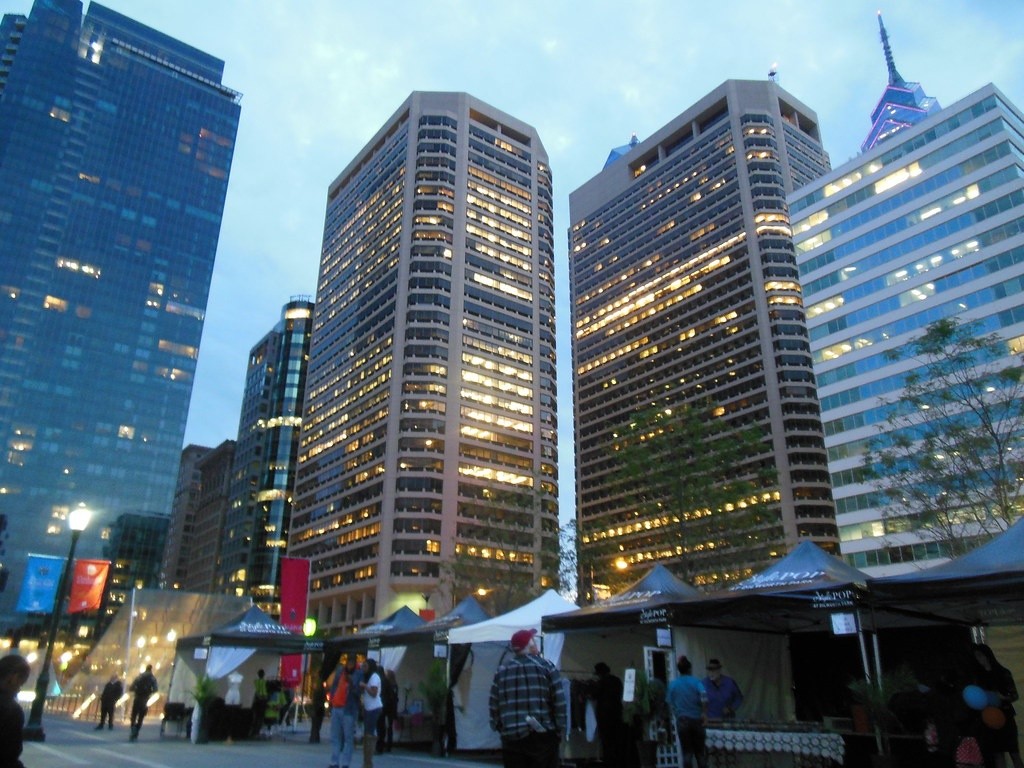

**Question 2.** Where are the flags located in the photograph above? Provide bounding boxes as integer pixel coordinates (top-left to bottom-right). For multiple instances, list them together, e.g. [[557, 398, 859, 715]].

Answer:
[[66, 558, 110, 613], [16, 553, 64, 615]]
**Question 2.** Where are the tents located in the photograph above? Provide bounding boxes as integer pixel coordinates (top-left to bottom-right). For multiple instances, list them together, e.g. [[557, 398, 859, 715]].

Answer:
[[164, 604, 323, 743], [327, 512, 1024, 768]]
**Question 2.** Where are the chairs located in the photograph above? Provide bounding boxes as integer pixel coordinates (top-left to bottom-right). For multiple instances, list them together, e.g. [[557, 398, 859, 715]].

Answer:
[[160, 702, 185, 737], [259, 688, 295, 741]]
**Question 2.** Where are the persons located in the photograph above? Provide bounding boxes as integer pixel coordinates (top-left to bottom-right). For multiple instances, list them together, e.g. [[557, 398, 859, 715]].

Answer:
[[586, 662, 624, 768], [126, 664, 158, 743], [253, 668, 287, 738], [93, 672, 124, 732], [701, 658, 744, 720], [488, 628, 567, 768], [974, 643, 1024, 768], [665, 655, 708, 768], [304, 678, 326, 745], [0, 654, 31, 767], [325, 651, 399, 768]]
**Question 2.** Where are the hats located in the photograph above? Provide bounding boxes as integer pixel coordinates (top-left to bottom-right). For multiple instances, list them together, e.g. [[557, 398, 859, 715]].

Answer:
[[677, 655, 691, 673], [512, 628, 538, 653], [705, 659, 722, 671]]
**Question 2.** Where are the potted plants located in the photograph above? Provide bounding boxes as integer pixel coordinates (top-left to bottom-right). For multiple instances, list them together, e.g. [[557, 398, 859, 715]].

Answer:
[[184, 672, 227, 743]]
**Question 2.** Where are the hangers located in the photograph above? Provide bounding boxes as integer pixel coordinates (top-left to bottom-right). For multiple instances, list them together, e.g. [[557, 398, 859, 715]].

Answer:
[[563, 670, 596, 681]]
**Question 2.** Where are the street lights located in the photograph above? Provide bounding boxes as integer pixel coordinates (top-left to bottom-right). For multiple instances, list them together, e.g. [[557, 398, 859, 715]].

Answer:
[[20, 501, 94, 744]]
